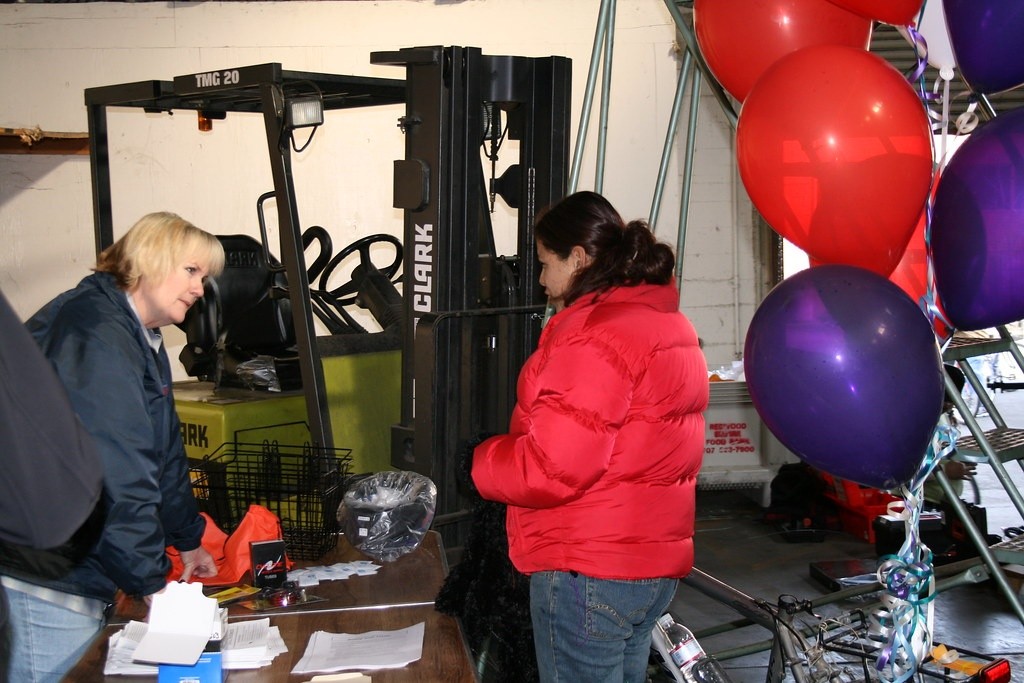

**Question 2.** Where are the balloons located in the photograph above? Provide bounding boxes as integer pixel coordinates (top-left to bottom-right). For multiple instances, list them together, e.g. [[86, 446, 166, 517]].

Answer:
[[691, 0, 1024, 492]]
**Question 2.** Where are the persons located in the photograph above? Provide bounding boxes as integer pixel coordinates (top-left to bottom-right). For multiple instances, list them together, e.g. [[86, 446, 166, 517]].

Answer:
[[0, 211, 226, 683], [474, 192, 709, 682]]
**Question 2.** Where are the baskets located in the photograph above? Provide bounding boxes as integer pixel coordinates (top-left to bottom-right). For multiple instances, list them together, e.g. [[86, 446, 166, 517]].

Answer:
[[189, 438, 357, 560]]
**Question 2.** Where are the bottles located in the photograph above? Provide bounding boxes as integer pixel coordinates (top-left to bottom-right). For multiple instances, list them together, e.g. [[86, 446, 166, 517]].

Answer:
[[660, 614, 724, 683]]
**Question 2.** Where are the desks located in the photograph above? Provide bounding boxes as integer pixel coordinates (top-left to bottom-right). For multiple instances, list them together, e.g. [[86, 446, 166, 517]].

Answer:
[[58, 531, 481, 682]]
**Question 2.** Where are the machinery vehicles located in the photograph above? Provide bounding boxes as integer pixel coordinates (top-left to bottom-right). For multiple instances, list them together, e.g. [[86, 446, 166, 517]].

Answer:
[[83, 43, 717, 683]]
